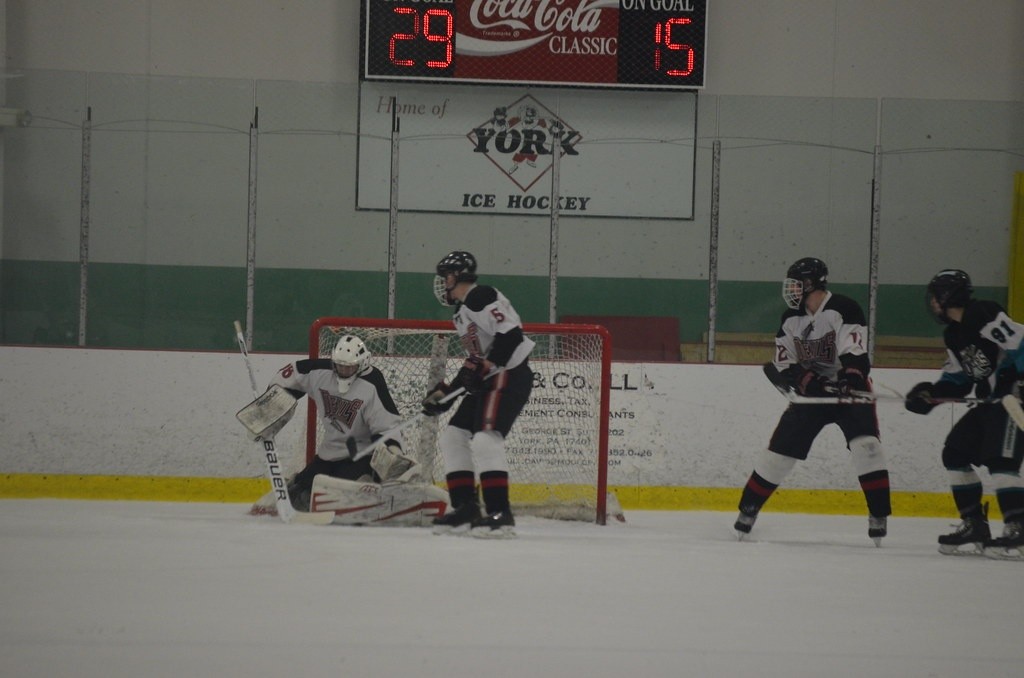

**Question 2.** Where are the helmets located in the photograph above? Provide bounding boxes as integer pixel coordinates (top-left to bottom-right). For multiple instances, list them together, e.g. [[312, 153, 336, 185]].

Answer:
[[331, 334, 371, 393], [927, 269, 972, 324], [433, 251, 478, 307], [782, 257, 828, 312]]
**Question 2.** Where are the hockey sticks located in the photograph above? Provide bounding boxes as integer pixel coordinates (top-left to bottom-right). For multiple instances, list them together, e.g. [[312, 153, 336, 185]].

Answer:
[[823, 383, 904, 400], [345, 363, 503, 462], [772, 372, 1024, 405], [232, 318, 335, 524]]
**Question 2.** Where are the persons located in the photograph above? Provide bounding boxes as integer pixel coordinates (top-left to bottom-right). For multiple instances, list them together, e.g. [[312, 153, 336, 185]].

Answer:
[[418, 250, 536, 540], [236, 335, 454, 527], [733, 258, 892, 546], [905, 270, 1024, 560]]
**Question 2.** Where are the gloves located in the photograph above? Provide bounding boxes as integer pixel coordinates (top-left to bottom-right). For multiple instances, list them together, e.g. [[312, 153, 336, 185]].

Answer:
[[421, 383, 453, 416], [837, 367, 876, 405], [457, 355, 486, 394], [905, 382, 938, 415], [790, 364, 827, 397]]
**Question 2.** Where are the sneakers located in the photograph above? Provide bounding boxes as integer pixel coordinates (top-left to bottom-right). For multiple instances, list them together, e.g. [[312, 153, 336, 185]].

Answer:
[[432, 506, 482, 536], [982, 513, 1024, 561], [734, 511, 757, 541], [471, 508, 518, 539], [868, 512, 887, 548], [938, 501, 992, 556]]
